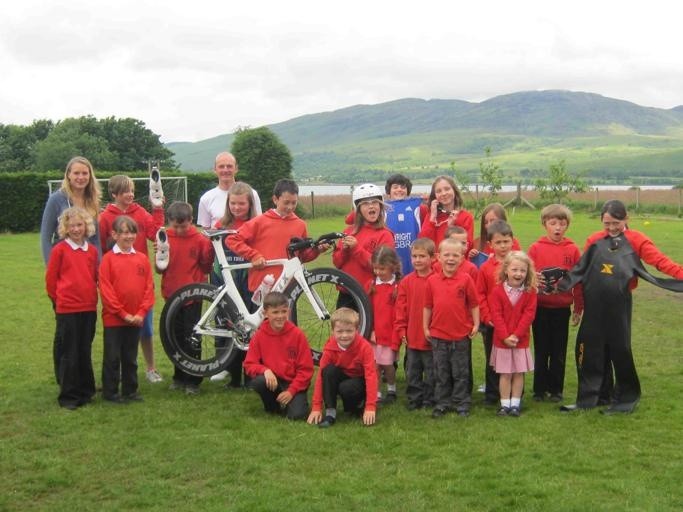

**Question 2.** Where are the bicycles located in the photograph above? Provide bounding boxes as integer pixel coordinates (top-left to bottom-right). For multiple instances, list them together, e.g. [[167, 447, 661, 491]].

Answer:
[[160, 230, 373, 378]]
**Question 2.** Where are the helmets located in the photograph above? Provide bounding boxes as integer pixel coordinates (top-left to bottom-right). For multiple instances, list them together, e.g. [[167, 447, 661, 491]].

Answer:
[[352, 184, 382, 210]]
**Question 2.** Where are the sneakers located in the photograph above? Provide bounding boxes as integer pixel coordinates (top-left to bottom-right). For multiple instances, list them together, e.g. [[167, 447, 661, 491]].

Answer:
[[384, 391, 470, 419], [155, 227, 170, 270], [145, 368, 162, 384], [149, 168, 164, 208], [169, 381, 199, 395], [111, 393, 142, 404], [66, 404, 76, 410], [319, 416, 335, 428], [223, 383, 240, 388], [481, 398, 519, 417], [534, 391, 560, 403]]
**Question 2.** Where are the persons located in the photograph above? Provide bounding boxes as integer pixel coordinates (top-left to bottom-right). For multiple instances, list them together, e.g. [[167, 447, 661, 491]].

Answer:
[[42, 156, 102, 393], [96, 174, 164, 383], [243, 293, 314, 421], [225, 178, 330, 319], [394, 176, 537, 420], [195, 151, 262, 387], [154, 199, 213, 396], [333, 184, 396, 313], [525, 204, 583, 401], [98, 215, 153, 403], [306, 307, 379, 427], [45, 204, 98, 410], [552, 199, 681, 414], [344, 174, 426, 276], [361, 247, 404, 403]]
